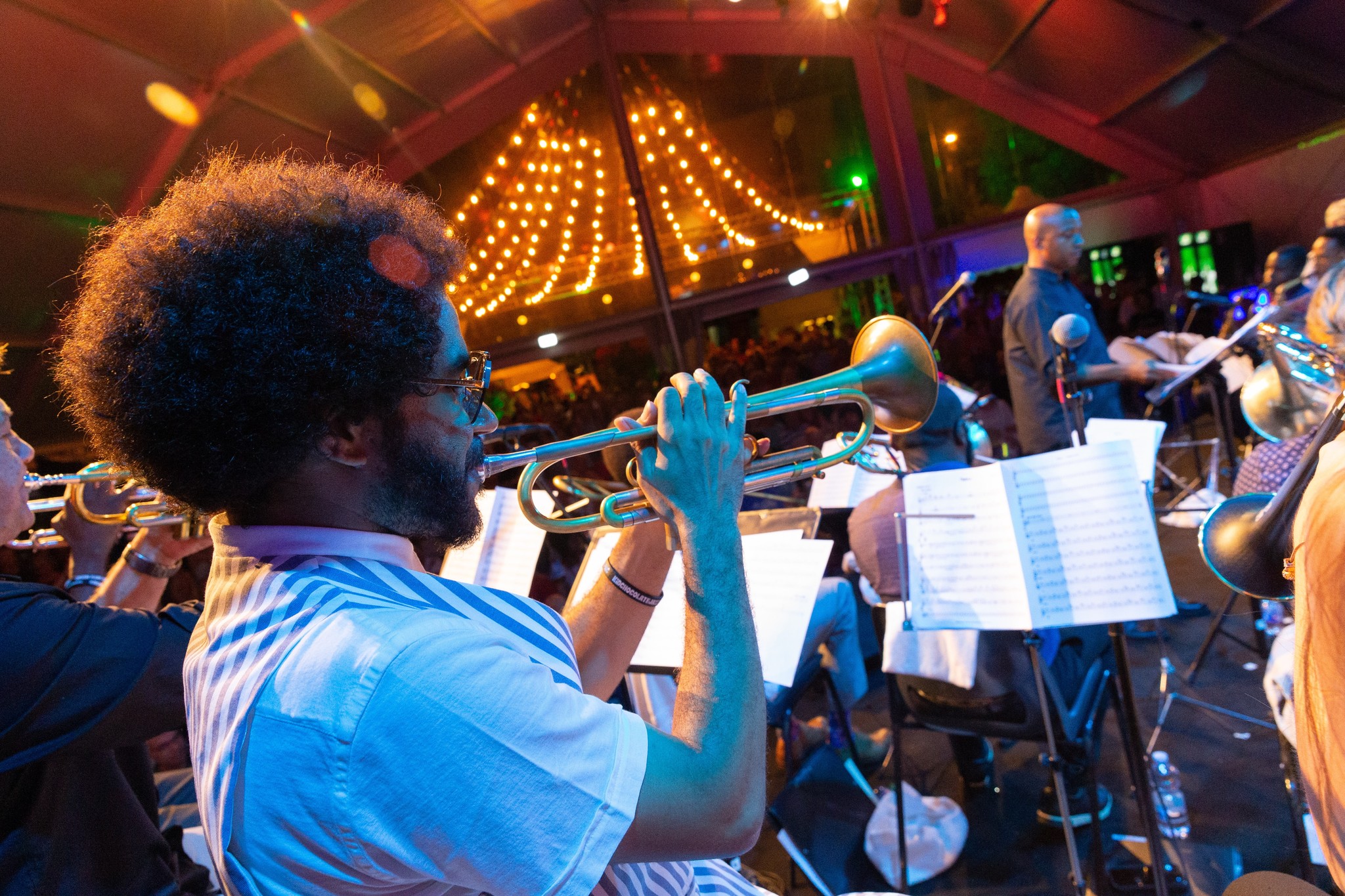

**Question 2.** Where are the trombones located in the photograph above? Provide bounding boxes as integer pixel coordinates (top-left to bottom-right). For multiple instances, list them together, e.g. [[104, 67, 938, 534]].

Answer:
[[834, 429, 1010, 477], [4, 459, 222, 554], [1240, 320, 1345, 442]]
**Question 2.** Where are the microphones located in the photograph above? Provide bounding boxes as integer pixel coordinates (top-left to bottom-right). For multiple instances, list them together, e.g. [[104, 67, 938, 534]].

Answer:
[[932, 270, 978, 316], [1182, 291, 1230, 306], [1049, 313, 1091, 349]]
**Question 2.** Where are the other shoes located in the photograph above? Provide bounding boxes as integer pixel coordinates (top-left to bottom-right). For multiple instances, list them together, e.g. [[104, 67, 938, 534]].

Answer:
[[840, 728, 893, 767], [791, 716, 830, 760], [1166, 600, 1211, 618], [1128, 628, 1168, 642]]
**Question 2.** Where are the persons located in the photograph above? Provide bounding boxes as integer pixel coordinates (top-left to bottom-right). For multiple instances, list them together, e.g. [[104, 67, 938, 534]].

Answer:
[[59, 151, 912, 896], [0, 343, 227, 896], [30, 201, 1345, 896]]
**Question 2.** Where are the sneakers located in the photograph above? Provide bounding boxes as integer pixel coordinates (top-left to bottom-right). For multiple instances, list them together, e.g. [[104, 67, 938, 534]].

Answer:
[[963, 737, 994, 786], [1034, 782, 1113, 826]]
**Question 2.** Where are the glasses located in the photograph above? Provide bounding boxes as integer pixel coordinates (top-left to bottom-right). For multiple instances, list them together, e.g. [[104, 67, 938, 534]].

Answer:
[[402, 350, 492, 425]]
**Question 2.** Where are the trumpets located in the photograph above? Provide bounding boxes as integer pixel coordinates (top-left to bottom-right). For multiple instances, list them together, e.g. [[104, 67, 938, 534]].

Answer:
[[1196, 371, 1342, 599], [469, 310, 940, 535]]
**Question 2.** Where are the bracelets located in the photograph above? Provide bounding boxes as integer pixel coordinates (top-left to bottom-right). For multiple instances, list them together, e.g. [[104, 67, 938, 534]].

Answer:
[[601, 558, 663, 609], [119, 543, 183, 579]]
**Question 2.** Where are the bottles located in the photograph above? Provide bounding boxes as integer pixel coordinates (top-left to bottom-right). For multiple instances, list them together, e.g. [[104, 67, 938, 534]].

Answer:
[[1260, 599, 1283, 646], [1149, 751, 1191, 840]]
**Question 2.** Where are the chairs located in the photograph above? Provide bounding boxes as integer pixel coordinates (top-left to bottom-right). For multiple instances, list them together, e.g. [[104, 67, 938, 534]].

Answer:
[[878, 612, 1135, 896], [622, 659, 874, 890]]
